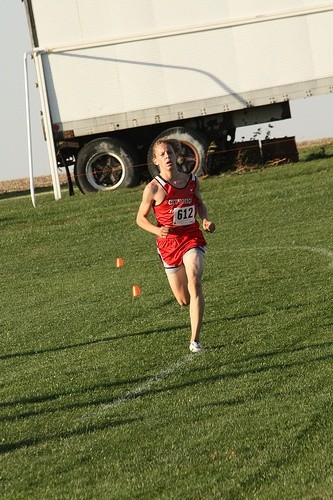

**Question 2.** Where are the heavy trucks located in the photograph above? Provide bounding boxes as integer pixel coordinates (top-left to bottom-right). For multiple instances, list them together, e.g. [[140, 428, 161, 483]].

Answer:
[[21, 5, 333, 209]]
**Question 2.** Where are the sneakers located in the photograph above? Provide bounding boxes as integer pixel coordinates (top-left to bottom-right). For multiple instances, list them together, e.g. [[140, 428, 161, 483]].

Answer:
[[189, 342, 202, 353]]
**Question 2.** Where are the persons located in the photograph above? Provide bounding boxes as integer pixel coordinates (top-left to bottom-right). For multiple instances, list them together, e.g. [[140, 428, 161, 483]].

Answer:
[[135, 140, 215, 354]]
